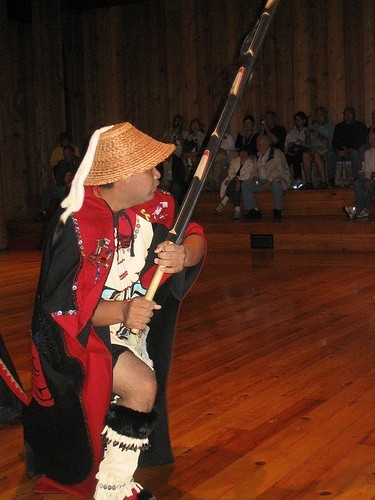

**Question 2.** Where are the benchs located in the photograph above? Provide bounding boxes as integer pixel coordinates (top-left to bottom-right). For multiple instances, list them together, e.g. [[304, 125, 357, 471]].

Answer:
[[156, 145, 375, 255], [6, 195, 49, 251]]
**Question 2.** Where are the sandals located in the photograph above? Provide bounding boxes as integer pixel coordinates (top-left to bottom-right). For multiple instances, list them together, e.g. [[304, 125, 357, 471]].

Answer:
[[315, 182, 328, 190], [299, 182, 314, 190]]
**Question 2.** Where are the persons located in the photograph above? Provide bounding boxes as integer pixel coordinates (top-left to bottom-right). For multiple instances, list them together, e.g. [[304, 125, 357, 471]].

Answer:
[[37, 170, 75, 223], [49, 130, 82, 185], [154, 142, 186, 212], [365, 109, 375, 151], [159, 106, 288, 194], [324, 105, 371, 190], [238, 132, 291, 223], [30, 121, 207, 500], [297, 106, 336, 192], [213, 144, 254, 220], [342, 130, 375, 221], [283, 111, 314, 191], [52, 144, 83, 186]]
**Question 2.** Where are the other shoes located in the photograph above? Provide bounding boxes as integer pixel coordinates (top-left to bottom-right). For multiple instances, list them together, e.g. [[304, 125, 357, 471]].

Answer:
[[342, 206, 356, 221], [272, 209, 282, 220], [244, 209, 261, 220], [214, 203, 226, 216], [233, 211, 241, 220], [357, 208, 369, 218]]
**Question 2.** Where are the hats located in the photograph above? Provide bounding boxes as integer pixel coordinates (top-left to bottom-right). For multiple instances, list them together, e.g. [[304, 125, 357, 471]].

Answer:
[[84, 122, 176, 187]]
